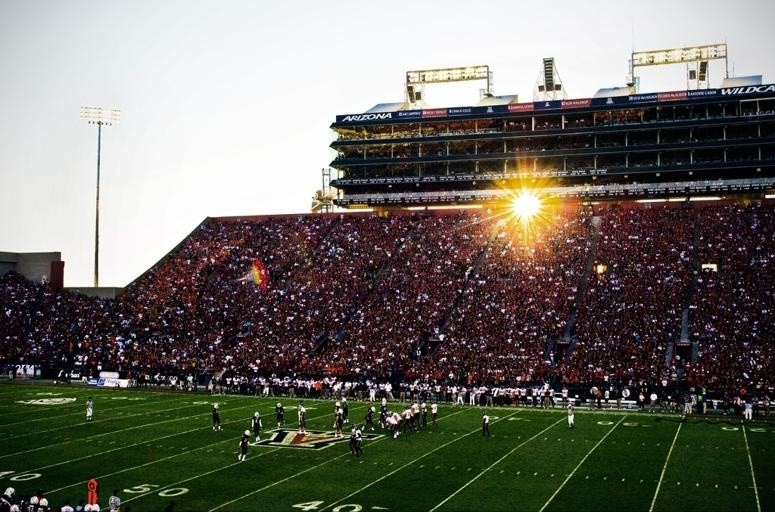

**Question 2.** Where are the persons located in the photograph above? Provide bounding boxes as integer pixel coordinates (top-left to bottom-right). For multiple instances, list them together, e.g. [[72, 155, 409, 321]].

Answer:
[[210, 404, 221, 431], [84, 396, 93, 421], [237, 429, 251, 461], [162, 499, 176, 511], [128, 213, 331, 397], [251, 410, 263, 442], [108, 488, 121, 511], [0, 484, 102, 511], [298, 400, 308, 434], [329, 97, 773, 454], [0, 267, 126, 383], [275, 402, 285, 429]]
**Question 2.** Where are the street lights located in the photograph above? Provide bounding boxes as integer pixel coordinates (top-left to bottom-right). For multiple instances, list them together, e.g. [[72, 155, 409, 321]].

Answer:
[[78, 103, 121, 286]]
